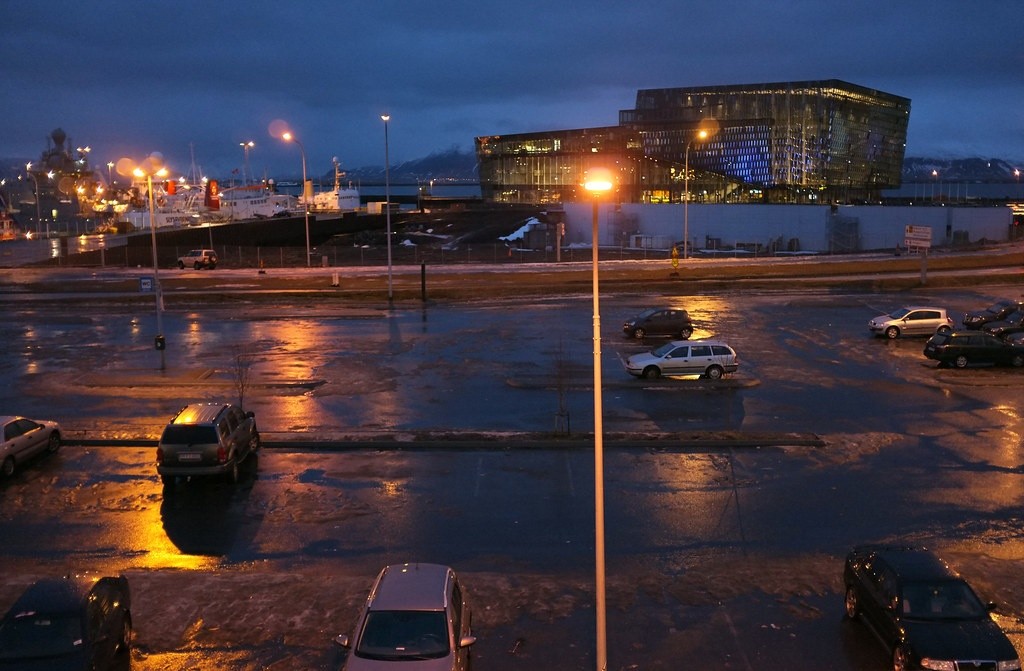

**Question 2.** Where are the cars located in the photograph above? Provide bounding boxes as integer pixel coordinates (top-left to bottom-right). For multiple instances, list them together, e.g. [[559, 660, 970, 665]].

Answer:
[[1003, 333, 1024, 348], [334, 561, 477, 671], [963, 299, 1024, 329], [0, 416, 63, 477], [0, 570, 134, 671], [868, 306, 954, 340], [981, 310, 1024, 336], [844, 541, 1022, 671], [622, 306, 693, 340]]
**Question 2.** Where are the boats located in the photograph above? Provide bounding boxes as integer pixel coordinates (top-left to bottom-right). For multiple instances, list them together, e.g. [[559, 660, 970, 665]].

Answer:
[[9, 121, 134, 234]]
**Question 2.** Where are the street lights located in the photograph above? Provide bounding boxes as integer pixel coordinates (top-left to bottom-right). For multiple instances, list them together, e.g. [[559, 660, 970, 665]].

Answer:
[[131, 160, 169, 341], [579, 169, 612, 669], [380, 113, 395, 300], [683, 130, 706, 261], [25, 171, 42, 240], [0, 177, 13, 210], [280, 130, 311, 269]]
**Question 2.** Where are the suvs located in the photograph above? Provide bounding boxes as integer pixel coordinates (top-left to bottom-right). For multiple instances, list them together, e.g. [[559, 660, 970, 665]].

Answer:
[[156, 402, 260, 484], [176, 248, 219, 272]]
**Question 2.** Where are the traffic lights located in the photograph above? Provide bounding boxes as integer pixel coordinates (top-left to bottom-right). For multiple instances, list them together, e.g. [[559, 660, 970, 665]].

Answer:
[[1014, 216, 1019, 226]]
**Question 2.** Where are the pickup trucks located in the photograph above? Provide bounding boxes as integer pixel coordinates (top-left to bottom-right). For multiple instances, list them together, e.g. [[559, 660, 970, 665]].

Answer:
[[923, 330, 1024, 369], [626, 340, 740, 380]]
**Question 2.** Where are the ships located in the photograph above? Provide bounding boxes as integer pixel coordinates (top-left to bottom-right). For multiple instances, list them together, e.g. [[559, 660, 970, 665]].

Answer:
[[95, 141, 364, 234]]
